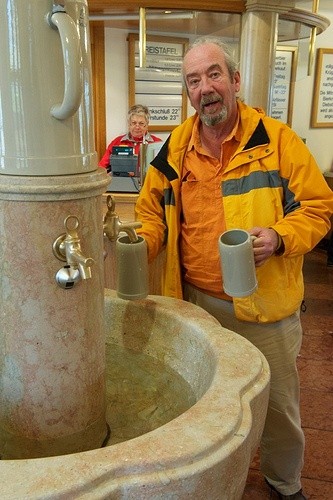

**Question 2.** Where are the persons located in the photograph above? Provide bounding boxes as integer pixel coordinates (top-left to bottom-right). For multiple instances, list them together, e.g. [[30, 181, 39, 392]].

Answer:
[[97, 104, 163, 178], [135, 38, 333, 500]]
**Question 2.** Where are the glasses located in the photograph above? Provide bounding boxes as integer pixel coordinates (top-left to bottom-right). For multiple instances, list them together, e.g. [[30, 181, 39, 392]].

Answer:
[[130, 121, 147, 127]]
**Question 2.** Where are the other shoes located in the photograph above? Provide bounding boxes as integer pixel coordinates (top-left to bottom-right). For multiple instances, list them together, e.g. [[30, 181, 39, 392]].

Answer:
[[264, 476, 307, 500]]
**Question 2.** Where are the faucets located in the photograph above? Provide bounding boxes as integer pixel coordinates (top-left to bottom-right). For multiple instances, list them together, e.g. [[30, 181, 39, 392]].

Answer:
[[105, 195, 143, 242], [64, 215, 96, 280]]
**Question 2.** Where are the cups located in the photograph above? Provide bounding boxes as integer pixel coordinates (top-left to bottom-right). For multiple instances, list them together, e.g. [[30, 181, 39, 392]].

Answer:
[[218, 229, 257, 297], [115, 235, 149, 300]]
